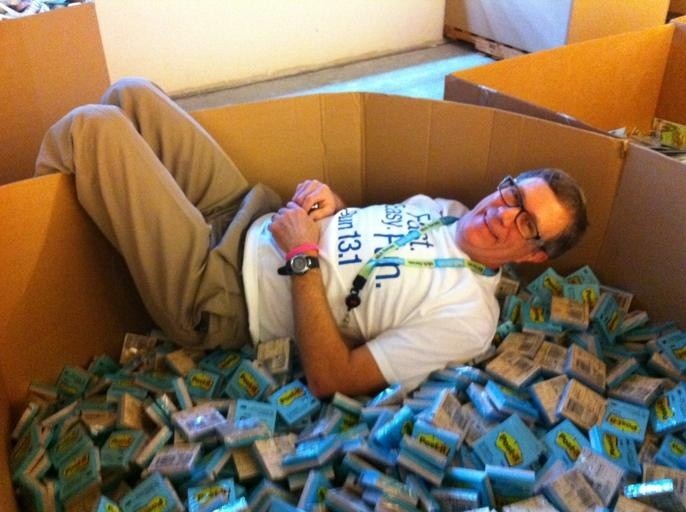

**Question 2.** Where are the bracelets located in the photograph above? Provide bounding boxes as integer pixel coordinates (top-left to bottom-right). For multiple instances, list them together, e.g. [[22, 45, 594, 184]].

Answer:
[[281, 242, 322, 258]]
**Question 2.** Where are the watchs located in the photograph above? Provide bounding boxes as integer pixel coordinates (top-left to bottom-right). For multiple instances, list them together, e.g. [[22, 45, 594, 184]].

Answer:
[[276, 256, 322, 278]]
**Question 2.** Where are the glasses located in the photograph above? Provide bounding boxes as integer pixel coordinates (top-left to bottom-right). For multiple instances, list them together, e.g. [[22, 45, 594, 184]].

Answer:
[[497, 174, 551, 257]]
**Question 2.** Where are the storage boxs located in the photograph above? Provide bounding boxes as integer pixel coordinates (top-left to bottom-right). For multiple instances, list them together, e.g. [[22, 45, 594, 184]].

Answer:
[[443, 23, 685, 165], [1, 2, 113, 184], [441, 0, 674, 61], [0, 85, 685, 510]]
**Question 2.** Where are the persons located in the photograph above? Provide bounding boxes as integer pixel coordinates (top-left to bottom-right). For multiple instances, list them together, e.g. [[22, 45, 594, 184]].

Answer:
[[33, 76, 592, 402]]
